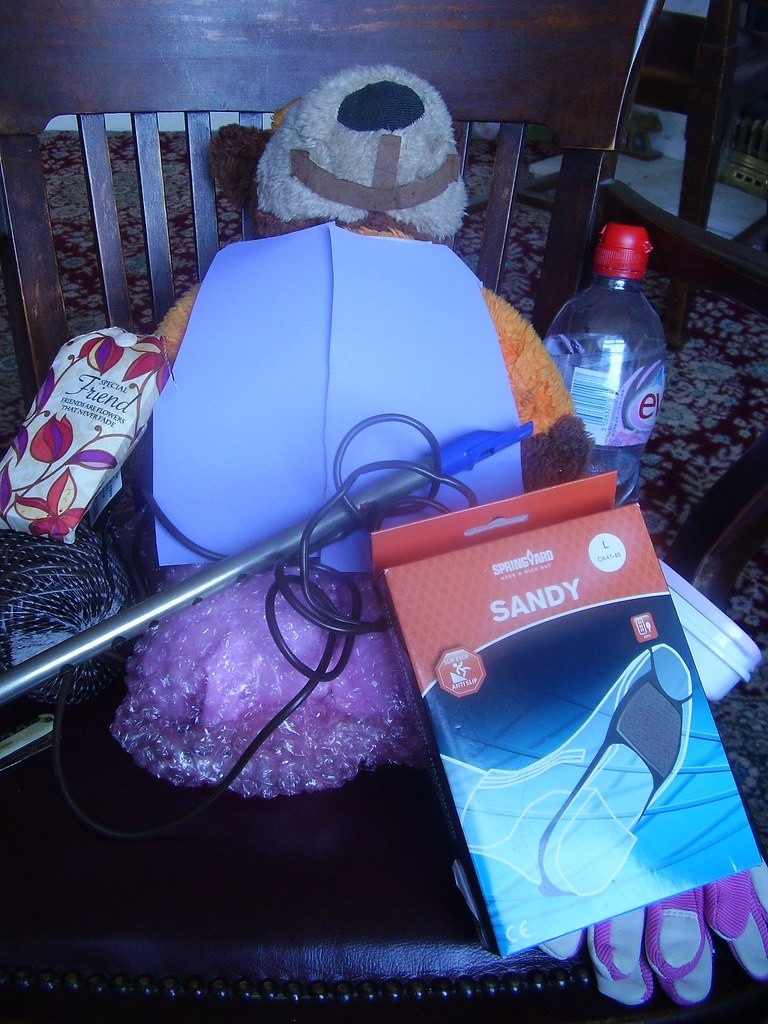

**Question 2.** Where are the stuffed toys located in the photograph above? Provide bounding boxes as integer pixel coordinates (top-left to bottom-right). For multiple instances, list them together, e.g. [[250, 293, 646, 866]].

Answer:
[[152, 62, 590, 494]]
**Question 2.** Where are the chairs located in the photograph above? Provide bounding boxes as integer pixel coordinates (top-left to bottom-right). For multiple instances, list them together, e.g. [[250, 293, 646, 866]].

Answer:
[[0, 0, 768, 1024]]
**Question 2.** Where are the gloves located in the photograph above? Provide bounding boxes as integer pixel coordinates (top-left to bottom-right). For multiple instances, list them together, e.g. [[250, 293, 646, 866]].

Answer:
[[536, 852, 768, 1005]]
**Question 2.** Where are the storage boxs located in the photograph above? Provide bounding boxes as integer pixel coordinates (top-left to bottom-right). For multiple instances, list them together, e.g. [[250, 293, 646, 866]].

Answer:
[[371, 468, 762, 961]]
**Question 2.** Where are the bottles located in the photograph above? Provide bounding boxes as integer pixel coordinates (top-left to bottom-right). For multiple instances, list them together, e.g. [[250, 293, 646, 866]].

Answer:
[[542, 222, 667, 508]]
